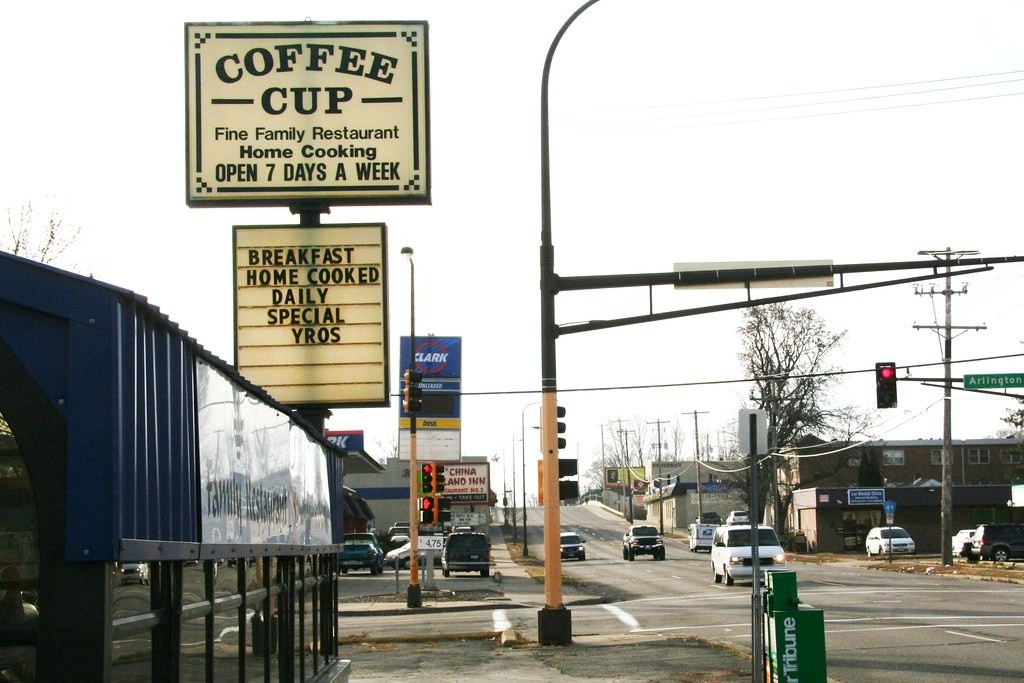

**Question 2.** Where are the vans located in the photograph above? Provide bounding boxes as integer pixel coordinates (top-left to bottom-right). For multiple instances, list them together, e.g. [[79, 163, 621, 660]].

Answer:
[[711, 523, 787, 585], [384, 535, 442, 570]]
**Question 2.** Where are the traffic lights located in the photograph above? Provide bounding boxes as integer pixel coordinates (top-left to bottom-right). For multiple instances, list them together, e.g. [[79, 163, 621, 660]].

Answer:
[[420, 497, 434, 524], [403, 368, 423, 414], [431, 463, 445, 494], [420, 463, 433, 494], [558, 458, 578, 501], [557, 405, 567, 449], [874, 362, 897, 409]]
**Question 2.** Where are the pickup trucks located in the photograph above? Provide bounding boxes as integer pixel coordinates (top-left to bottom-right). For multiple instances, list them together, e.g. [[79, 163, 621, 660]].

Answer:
[[338, 532, 384, 576]]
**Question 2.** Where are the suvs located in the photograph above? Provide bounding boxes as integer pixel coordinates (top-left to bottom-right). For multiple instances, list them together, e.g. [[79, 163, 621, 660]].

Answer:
[[440, 533, 493, 578], [623, 526, 665, 561], [970, 524, 1024, 563]]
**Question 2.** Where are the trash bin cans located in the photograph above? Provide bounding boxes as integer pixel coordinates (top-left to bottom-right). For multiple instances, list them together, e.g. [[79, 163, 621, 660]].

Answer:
[[759, 567, 829, 683]]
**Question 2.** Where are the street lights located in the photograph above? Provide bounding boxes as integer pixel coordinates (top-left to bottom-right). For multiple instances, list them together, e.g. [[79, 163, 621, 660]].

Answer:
[[400, 247, 423, 609]]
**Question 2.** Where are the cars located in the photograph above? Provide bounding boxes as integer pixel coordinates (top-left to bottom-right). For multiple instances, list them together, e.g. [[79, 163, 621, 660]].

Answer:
[[952, 529, 977, 556], [695, 511, 722, 524], [864, 528, 917, 558], [368, 521, 472, 555], [181, 558, 198, 567], [219, 558, 258, 567], [121, 562, 150, 586], [560, 532, 586, 562], [726, 510, 749, 525]]
